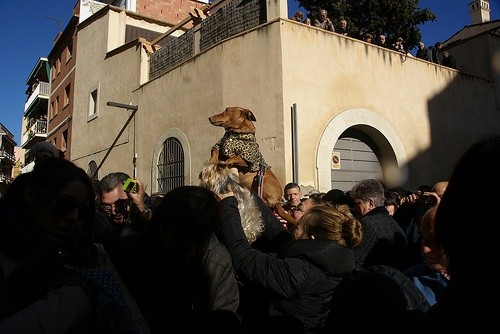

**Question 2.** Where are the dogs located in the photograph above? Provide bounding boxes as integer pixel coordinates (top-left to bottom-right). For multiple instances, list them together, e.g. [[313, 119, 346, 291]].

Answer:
[[199, 107, 299, 246]]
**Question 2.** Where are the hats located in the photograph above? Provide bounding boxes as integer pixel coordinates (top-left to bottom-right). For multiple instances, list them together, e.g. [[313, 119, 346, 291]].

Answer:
[[323, 189, 346, 203], [423, 180, 461, 200]]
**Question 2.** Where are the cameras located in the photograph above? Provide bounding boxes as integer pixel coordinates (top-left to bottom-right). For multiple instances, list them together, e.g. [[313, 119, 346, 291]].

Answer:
[[123, 178, 137, 193]]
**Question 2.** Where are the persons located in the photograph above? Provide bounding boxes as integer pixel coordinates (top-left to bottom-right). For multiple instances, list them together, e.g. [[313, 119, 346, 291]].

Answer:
[[288, 9, 457, 69], [0, 135, 500, 334]]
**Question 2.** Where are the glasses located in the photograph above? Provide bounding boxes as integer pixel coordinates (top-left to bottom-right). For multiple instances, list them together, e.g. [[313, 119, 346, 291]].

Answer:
[[52, 195, 91, 217], [101, 204, 129, 215]]
